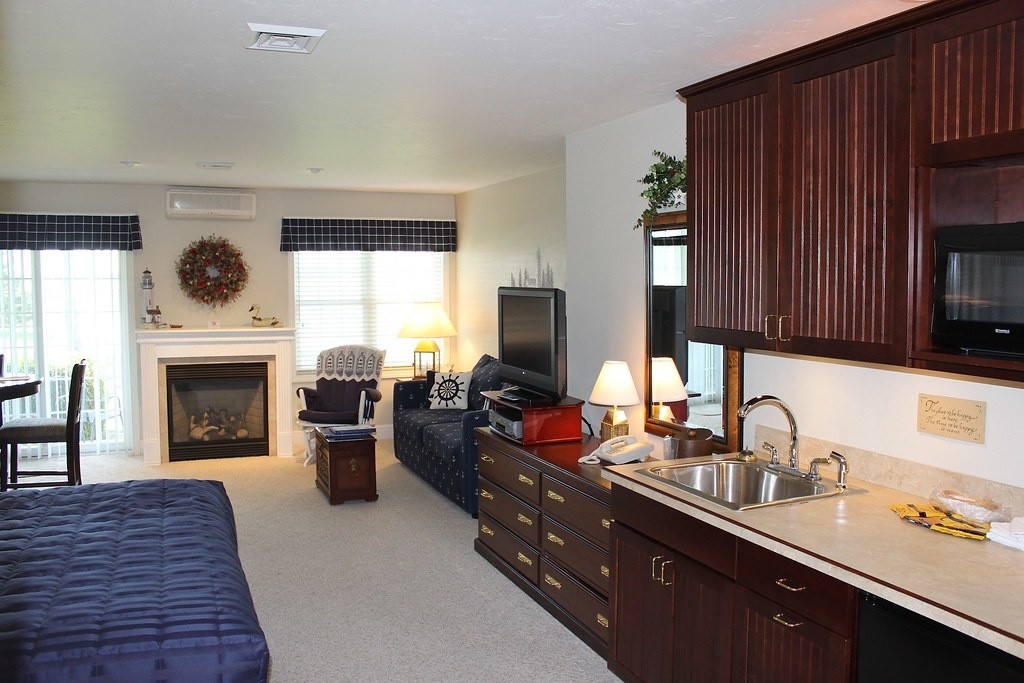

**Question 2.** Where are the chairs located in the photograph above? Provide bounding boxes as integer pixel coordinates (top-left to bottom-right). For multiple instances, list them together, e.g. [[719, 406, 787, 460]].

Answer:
[[0, 359, 87, 487], [297, 345, 386, 472]]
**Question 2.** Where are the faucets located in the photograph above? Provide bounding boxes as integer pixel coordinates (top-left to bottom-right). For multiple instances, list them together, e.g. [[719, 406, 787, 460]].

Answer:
[[829, 450, 850, 489], [737, 394, 833, 481]]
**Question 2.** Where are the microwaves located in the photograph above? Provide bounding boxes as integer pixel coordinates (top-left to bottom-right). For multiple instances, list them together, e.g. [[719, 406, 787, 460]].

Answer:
[[929, 222, 1024, 360]]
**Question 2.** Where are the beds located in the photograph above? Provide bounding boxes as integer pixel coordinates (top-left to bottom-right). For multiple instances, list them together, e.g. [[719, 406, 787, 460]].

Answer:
[[0, 479, 271, 683]]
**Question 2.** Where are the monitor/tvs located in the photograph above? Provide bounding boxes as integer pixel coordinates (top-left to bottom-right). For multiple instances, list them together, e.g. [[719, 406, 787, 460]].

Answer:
[[930, 222, 1024, 358], [498, 287, 568, 406]]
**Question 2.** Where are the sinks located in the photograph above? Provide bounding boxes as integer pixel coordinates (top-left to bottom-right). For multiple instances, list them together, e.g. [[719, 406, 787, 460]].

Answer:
[[633, 456, 869, 512]]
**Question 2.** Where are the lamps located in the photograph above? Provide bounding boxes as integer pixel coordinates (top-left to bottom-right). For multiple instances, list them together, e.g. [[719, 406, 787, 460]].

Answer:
[[397, 302, 455, 378], [587, 360, 641, 441], [140, 269, 155, 319]]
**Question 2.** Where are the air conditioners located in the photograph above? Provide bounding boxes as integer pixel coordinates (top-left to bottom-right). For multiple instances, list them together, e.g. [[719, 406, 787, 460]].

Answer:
[[165, 190, 256, 221]]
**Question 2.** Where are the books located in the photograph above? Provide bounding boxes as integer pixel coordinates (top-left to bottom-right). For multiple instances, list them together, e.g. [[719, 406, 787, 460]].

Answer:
[[322, 425, 377, 439]]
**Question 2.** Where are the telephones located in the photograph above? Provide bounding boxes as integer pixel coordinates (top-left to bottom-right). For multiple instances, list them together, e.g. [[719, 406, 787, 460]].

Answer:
[[594, 435, 655, 464]]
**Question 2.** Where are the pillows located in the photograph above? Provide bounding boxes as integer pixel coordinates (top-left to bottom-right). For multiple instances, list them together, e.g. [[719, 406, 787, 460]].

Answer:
[[429, 372, 473, 409], [467, 353, 504, 411]]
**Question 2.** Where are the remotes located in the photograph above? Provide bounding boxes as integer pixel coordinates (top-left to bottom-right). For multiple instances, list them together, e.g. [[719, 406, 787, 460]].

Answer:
[[497, 394, 521, 401]]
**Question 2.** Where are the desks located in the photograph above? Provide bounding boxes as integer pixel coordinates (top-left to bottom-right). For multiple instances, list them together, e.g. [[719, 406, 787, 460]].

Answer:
[[0, 375, 41, 401]]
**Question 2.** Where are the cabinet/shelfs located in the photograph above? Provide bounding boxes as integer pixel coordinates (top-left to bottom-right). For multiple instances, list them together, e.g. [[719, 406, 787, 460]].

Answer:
[[676, 0, 1024, 383], [313, 428, 377, 505], [473, 390, 860, 683]]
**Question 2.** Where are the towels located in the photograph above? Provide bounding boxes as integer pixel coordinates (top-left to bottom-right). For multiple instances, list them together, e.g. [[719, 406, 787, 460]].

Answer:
[[985, 516, 1024, 552]]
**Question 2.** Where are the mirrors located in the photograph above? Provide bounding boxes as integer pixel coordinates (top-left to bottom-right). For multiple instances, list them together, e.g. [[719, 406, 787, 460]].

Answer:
[[642, 211, 745, 452], [651, 357, 689, 420]]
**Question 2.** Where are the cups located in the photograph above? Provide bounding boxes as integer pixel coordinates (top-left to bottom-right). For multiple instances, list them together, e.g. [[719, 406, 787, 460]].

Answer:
[[666, 431, 712, 458]]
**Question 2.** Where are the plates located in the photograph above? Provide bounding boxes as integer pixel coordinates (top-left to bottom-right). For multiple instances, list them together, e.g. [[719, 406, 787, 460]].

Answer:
[[938, 497, 1006, 522]]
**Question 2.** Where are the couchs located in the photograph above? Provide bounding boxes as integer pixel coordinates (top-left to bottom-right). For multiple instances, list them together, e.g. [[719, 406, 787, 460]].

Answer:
[[393, 380, 491, 518]]
